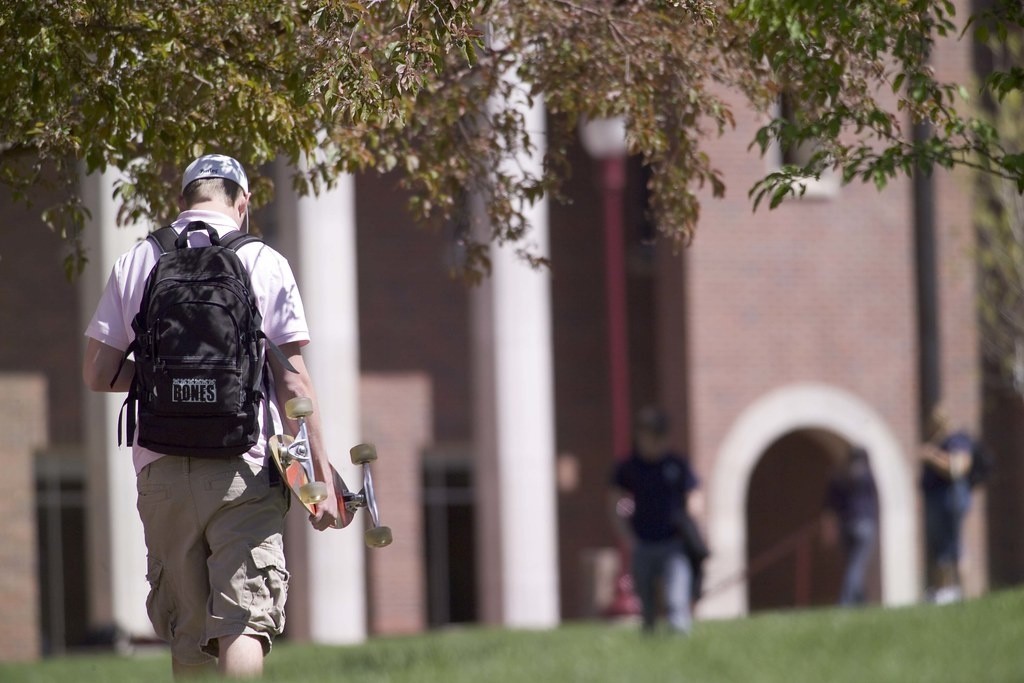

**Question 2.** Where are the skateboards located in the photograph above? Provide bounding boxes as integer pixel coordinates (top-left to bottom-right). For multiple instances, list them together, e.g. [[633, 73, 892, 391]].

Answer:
[[268, 397, 393, 551]]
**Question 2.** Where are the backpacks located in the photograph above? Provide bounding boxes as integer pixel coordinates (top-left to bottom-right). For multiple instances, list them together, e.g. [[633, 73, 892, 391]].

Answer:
[[111, 222, 300, 458]]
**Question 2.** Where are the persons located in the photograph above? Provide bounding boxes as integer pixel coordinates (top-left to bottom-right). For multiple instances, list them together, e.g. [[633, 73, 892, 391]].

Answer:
[[82, 152, 342, 683], [915, 398, 973, 607], [608, 412, 710, 636], [824, 447, 880, 610]]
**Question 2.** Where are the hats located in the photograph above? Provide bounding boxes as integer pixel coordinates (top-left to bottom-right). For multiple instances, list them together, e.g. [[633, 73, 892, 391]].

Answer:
[[181, 153, 249, 196]]
